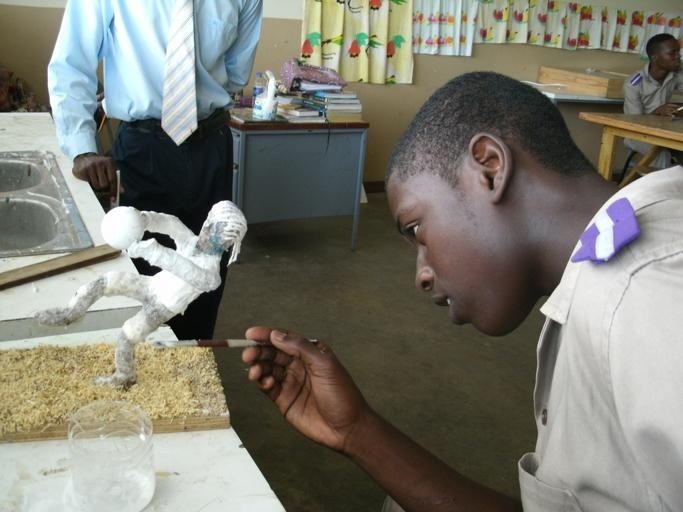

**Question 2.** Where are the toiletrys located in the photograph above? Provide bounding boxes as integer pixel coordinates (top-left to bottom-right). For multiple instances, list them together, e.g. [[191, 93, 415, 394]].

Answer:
[[252, 69, 279, 122]]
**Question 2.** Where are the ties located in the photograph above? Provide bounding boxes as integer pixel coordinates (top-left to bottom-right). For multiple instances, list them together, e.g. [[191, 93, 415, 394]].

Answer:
[[161, 1, 199, 149]]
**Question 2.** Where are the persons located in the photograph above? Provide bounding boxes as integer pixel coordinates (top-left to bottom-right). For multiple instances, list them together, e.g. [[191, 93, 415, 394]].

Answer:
[[44, 0, 265, 342], [236, 68, 682, 512], [34, 194, 247, 390], [618, 33, 683, 170]]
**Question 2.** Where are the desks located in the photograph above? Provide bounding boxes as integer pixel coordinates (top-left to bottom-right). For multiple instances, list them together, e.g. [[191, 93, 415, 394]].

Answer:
[[525, 81, 683, 188]]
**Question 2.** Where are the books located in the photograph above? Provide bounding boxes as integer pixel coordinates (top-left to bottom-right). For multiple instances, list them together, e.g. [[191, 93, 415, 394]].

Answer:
[[274, 80, 364, 126]]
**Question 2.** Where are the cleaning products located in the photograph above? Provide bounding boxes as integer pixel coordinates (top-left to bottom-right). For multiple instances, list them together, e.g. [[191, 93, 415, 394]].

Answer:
[[252, 72, 266, 107]]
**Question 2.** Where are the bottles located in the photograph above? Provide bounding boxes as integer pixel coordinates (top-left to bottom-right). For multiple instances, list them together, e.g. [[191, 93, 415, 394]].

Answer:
[[250, 71, 266, 107]]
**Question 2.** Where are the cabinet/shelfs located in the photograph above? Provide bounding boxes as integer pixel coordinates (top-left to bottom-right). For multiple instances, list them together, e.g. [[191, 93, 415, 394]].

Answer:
[[227, 98, 370, 251]]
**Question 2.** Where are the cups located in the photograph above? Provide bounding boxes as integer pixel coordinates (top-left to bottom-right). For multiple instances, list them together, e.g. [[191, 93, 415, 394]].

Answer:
[[68, 401, 156, 512]]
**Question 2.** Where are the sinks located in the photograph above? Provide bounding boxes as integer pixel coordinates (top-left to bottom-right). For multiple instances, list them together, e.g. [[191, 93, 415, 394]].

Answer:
[[0, 195, 95, 256], [0, 150, 62, 194]]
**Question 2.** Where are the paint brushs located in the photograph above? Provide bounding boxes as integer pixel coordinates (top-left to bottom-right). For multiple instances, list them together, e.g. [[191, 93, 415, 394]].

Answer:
[[154, 339, 319, 347]]
